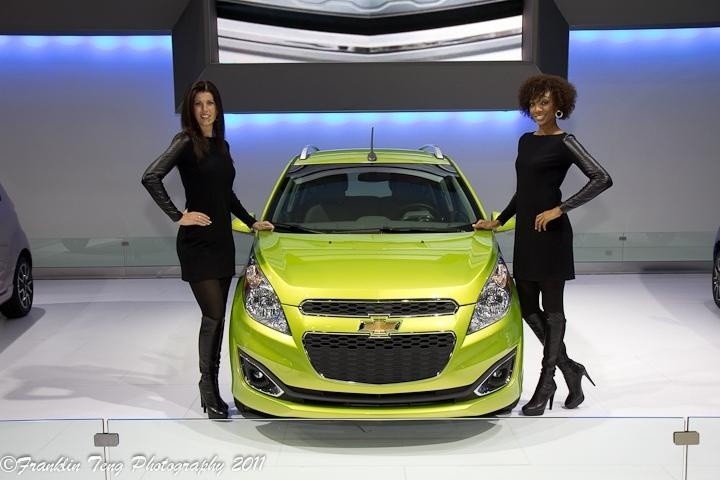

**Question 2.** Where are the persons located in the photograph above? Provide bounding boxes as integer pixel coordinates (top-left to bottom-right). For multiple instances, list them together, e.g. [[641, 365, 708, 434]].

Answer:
[[472, 75, 613, 416], [140, 80, 275, 419]]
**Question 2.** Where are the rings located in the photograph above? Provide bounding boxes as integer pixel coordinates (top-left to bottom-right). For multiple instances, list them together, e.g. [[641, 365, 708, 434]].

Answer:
[[198, 215, 200, 219]]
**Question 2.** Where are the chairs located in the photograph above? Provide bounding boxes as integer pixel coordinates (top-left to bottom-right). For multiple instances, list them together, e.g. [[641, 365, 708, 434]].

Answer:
[[296, 173, 432, 224]]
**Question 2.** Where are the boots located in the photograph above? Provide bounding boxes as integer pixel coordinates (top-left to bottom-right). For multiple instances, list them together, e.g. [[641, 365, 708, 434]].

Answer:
[[523, 308, 595, 416], [199, 315, 228, 418]]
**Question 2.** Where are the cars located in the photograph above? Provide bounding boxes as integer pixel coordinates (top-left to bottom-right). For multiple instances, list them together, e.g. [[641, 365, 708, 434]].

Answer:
[[0, 184, 35, 321], [228, 124, 525, 424]]
